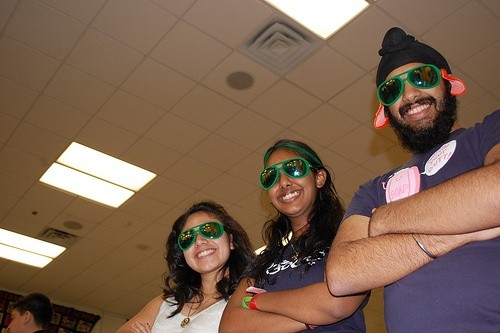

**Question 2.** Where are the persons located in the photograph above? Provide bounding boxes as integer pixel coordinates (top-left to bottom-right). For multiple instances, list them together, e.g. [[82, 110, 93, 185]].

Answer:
[[5, 293, 54, 333], [115, 201, 257, 333], [324, 26, 500, 333], [217, 140, 376, 333]]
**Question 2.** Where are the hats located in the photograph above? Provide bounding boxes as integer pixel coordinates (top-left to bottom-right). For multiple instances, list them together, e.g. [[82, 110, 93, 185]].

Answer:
[[371, 27, 465, 129]]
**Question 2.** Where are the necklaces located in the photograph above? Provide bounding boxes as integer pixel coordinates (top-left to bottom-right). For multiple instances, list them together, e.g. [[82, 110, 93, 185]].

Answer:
[[180, 287, 219, 328]]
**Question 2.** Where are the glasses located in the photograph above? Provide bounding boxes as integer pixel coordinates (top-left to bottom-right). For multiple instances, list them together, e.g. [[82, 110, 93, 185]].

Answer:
[[259, 158, 319, 191], [178, 221, 231, 252], [377, 64, 441, 105]]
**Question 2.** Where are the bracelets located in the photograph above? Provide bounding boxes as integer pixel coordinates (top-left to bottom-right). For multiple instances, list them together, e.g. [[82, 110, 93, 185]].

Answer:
[[250, 293, 263, 310], [412, 234, 439, 260], [242, 297, 253, 310]]
